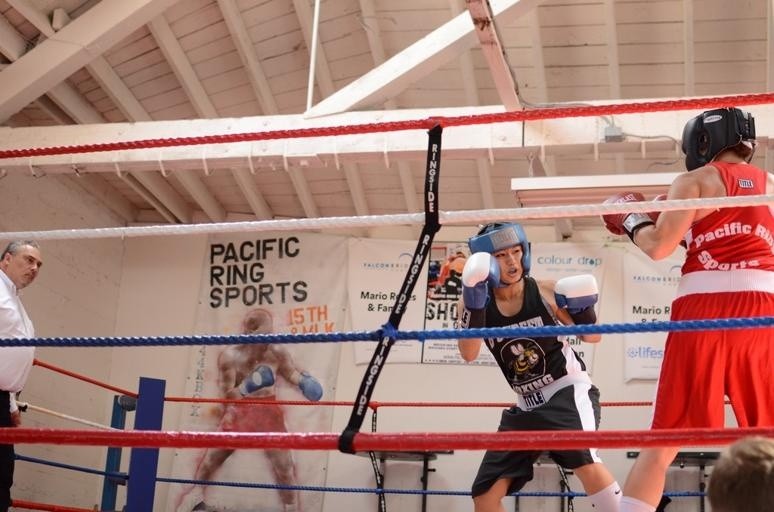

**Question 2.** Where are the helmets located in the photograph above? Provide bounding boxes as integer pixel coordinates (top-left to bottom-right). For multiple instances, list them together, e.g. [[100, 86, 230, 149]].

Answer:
[[681, 107, 758, 171], [467, 221, 531, 288]]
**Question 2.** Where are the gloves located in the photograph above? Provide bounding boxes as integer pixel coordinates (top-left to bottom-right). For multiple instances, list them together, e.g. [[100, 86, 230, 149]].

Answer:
[[600, 191, 655, 246], [461, 251, 501, 329], [553, 274, 599, 325], [238, 364, 275, 399], [648, 194, 687, 250], [298, 370, 323, 401]]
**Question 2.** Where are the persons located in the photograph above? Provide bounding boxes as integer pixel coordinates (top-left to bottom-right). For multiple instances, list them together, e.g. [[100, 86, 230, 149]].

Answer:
[[175, 308, 325, 512], [0, 237, 46, 512], [704, 429, 774, 511], [454, 220, 625, 511], [597, 105, 774, 512]]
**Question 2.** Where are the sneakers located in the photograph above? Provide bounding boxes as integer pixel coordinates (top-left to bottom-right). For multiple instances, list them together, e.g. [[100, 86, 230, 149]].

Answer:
[[175, 493, 205, 512]]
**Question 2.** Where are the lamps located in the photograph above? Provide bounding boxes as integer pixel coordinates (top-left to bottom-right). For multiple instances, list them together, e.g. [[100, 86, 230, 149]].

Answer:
[[510, 158, 685, 208]]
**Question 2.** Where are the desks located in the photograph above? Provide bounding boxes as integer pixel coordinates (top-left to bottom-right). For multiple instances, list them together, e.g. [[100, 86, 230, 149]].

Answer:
[[515, 450, 573, 512], [355, 450, 453, 512], [627, 452, 720, 512]]
